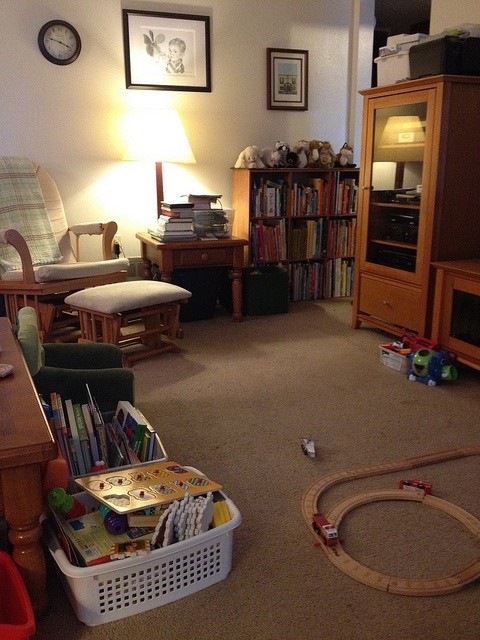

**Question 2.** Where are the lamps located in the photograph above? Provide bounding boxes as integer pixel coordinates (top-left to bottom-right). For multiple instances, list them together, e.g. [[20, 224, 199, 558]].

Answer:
[[116, 91, 198, 231]]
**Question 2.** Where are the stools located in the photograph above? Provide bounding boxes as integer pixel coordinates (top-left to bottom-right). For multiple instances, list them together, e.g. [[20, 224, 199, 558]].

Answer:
[[64, 280, 192, 366]]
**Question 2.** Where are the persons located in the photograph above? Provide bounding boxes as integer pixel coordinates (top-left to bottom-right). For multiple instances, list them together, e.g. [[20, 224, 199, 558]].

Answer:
[[122, 543, 134, 551], [164, 38, 187, 74]]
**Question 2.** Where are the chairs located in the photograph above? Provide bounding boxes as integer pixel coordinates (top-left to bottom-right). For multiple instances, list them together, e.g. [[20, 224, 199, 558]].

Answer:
[[21, 308, 134, 427], [0, 154, 128, 345]]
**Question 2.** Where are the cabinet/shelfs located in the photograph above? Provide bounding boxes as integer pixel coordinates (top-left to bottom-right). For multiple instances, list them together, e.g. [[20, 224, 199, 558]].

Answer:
[[429, 256, 480, 372], [230, 167, 361, 303], [350, 76, 480, 339]]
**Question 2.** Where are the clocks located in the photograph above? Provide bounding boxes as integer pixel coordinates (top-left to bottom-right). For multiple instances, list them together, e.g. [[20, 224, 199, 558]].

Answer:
[[37, 19, 81, 65]]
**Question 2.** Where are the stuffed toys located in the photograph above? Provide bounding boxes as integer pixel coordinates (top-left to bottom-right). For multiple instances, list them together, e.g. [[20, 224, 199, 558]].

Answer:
[[287, 151, 298, 168], [271, 140, 292, 168], [307, 140, 323, 168], [334, 142, 356, 168], [259, 147, 277, 168], [320, 141, 338, 169], [293, 140, 310, 168], [235, 145, 266, 169]]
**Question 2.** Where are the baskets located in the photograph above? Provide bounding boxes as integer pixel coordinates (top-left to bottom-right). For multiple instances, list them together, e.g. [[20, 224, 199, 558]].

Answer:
[[38, 466, 242, 627], [378, 340, 427, 375], [66, 407, 169, 496]]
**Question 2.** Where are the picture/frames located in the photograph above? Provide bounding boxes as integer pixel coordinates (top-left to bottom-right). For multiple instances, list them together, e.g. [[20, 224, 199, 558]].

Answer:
[[267, 47, 308, 109], [122, 9, 210, 92]]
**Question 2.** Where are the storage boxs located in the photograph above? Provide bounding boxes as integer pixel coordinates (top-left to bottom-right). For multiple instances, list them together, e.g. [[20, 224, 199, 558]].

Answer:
[[374, 51, 409, 86], [410, 36, 480, 76]]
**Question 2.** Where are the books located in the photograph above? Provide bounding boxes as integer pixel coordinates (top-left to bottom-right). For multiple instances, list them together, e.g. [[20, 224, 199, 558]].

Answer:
[[184, 193, 224, 208], [336, 173, 359, 215], [251, 176, 287, 218], [147, 200, 198, 246], [248, 219, 287, 263], [279, 258, 355, 303], [290, 172, 327, 217], [290, 218, 324, 260], [48, 382, 157, 475], [326, 217, 359, 258]]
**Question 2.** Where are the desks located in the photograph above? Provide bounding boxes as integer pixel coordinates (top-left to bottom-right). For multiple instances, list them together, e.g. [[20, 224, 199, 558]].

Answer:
[[1, 312, 62, 622], [136, 232, 247, 319]]
